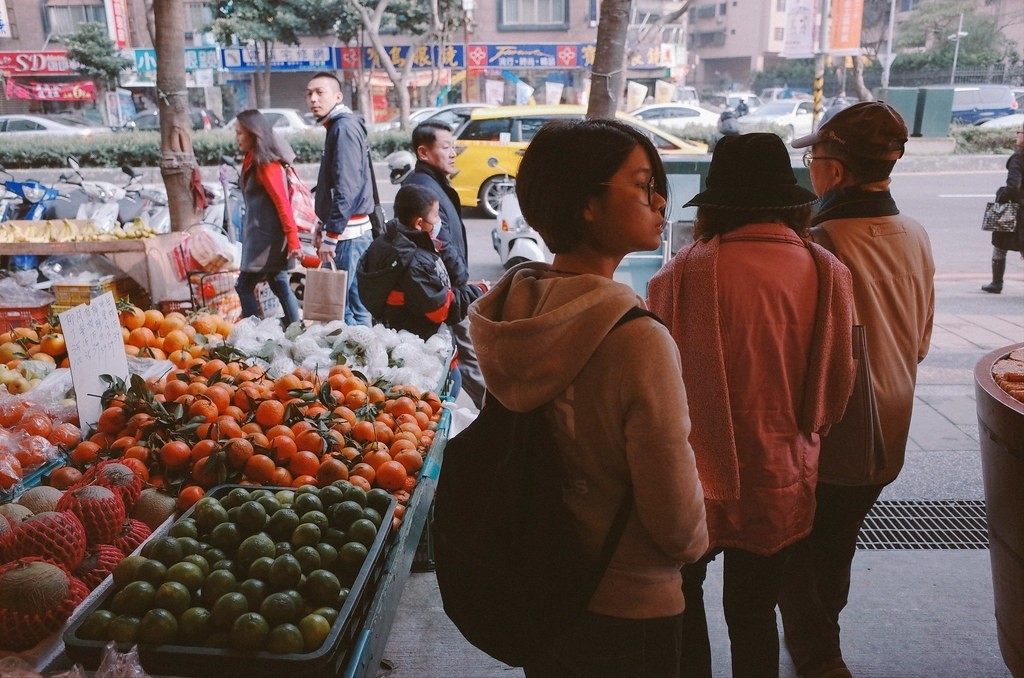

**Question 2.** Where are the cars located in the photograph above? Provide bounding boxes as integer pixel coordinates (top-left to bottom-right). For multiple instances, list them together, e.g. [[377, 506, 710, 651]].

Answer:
[[0, 112, 112, 148], [109, 107, 226, 133], [361, 87, 862, 218], [223, 107, 326, 131]]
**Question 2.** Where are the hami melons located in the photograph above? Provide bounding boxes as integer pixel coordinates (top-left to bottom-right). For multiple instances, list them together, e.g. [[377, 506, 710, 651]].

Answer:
[[0, 465, 178, 654]]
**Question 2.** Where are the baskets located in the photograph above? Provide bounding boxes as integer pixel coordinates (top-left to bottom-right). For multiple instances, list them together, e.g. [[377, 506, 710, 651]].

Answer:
[[63, 483, 397, 678]]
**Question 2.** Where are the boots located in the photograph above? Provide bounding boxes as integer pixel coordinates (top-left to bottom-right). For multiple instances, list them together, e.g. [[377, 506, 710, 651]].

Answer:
[[982, 258, 1005, 294]]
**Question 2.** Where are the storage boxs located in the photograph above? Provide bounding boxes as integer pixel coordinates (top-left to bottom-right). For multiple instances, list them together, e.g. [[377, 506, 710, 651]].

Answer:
[[57, 484, 398, 678], [51, 276, 132, 302], [0, 300, 55, 336]]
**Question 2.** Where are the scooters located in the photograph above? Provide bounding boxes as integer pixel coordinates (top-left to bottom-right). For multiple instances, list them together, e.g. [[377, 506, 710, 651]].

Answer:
[[0, 154, 324, 301], [491, 190, 555, 273]]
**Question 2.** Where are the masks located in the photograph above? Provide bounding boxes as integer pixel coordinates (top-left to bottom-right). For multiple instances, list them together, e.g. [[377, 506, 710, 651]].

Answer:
[[421, 218, 441, 239]]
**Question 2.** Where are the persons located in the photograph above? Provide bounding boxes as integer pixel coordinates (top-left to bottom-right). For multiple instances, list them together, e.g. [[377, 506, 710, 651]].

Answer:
[[233, 107, 302, 326], [643, 133, 858, 678], [355, 184, 492, 344], [792, 98, 933, 677], [981, 122, 1024, 293], [400, 121, 486, 414], [469, 121, 710, 678], [708, 112, 746, 155], [304, 72, 372, 330]]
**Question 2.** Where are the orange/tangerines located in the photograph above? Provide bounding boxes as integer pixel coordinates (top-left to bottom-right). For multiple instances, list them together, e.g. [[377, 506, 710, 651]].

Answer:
[[79, 480, 390, 657], [0, 305, 443, 530]]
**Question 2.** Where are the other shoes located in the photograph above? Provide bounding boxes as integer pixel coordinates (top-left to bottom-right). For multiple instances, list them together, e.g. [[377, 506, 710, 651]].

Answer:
[[284, 319, 305, 333], [799, 655, 852, 678]]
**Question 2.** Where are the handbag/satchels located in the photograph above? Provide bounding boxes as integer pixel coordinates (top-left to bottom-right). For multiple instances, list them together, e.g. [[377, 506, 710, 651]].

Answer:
[[982, 190, 1020, 232], [303, 257, 347, 322], [368, 204, 387, 241], [806, 227, 888, 486]]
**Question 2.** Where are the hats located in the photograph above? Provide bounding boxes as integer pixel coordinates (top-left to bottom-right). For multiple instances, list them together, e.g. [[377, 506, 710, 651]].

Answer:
[[791, 100, 908, 161], [682, 132, 820, 212]]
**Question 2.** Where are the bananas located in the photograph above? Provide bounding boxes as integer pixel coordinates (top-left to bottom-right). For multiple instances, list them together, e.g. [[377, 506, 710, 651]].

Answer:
[[0, 216, 157, 244]]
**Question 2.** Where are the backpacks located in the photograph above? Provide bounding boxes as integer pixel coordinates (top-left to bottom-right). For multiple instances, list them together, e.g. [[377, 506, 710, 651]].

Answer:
[[426, 308, 665, 667], [278, 160, 319, 233]]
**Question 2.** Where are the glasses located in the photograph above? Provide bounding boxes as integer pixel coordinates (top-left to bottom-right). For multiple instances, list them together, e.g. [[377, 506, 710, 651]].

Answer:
[[1017, 131, 1024, 134], [597, 176, 655, 205], [803, 151, 848, 169]]
